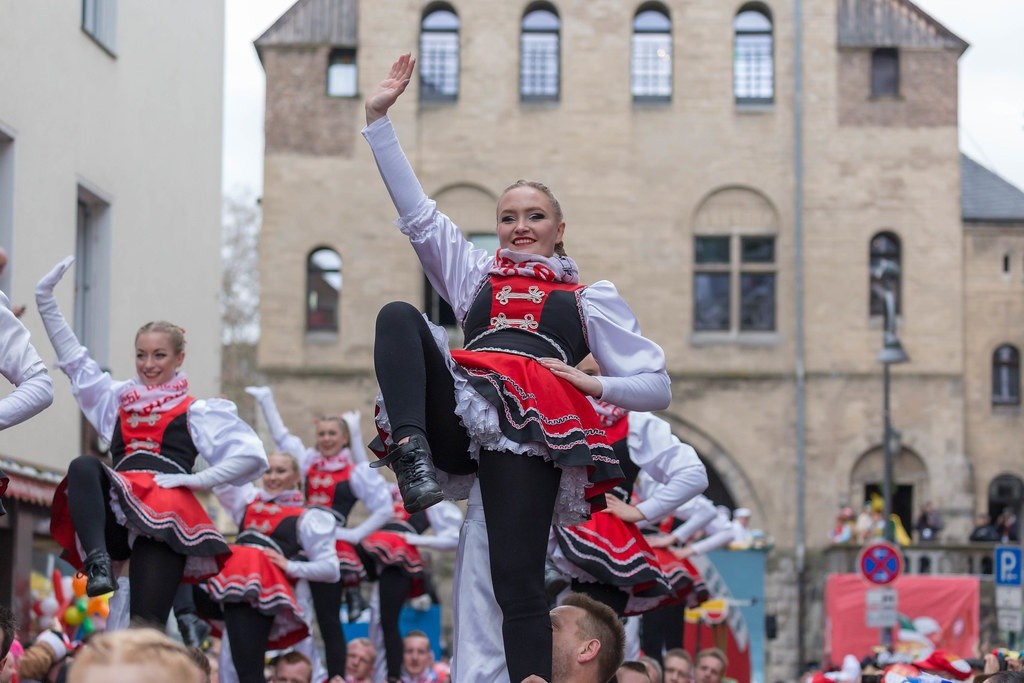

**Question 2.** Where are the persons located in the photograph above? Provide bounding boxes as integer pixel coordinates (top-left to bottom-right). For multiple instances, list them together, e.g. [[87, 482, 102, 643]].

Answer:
[[0, 251, 463, 683], [450, 478, 625, 683], [970, 506, 1018, 574], [798, 647, 1024, 683], [545, 352, 774, 683], [916, 502, 944, 572], [830, 497, 911, 573], [361, 52, 671, 683]]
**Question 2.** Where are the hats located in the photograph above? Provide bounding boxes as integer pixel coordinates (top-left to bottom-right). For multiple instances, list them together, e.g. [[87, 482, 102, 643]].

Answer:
[[913, 617, 939, 636], [734, 508, 751, 517]]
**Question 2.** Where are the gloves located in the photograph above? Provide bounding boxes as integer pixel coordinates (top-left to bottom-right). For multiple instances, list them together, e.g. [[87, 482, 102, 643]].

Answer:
[[406, 529, 458, 550], [0, 372, 54, 432], [35, 256, 88, 367], [244, 386, 288, 442], [342, 410, 367, 465], [341, 505, 392, 545], [152, 456, 261, 491]]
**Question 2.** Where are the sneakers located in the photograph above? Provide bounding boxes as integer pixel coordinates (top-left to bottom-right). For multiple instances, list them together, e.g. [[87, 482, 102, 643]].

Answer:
[[369, 434, 445, 514], [177, 613, 211, 648], [76, 550, 119, 597]]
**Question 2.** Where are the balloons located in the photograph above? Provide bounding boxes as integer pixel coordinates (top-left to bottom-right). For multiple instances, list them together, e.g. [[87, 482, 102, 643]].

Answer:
[[30, 571, 114, 643]]
